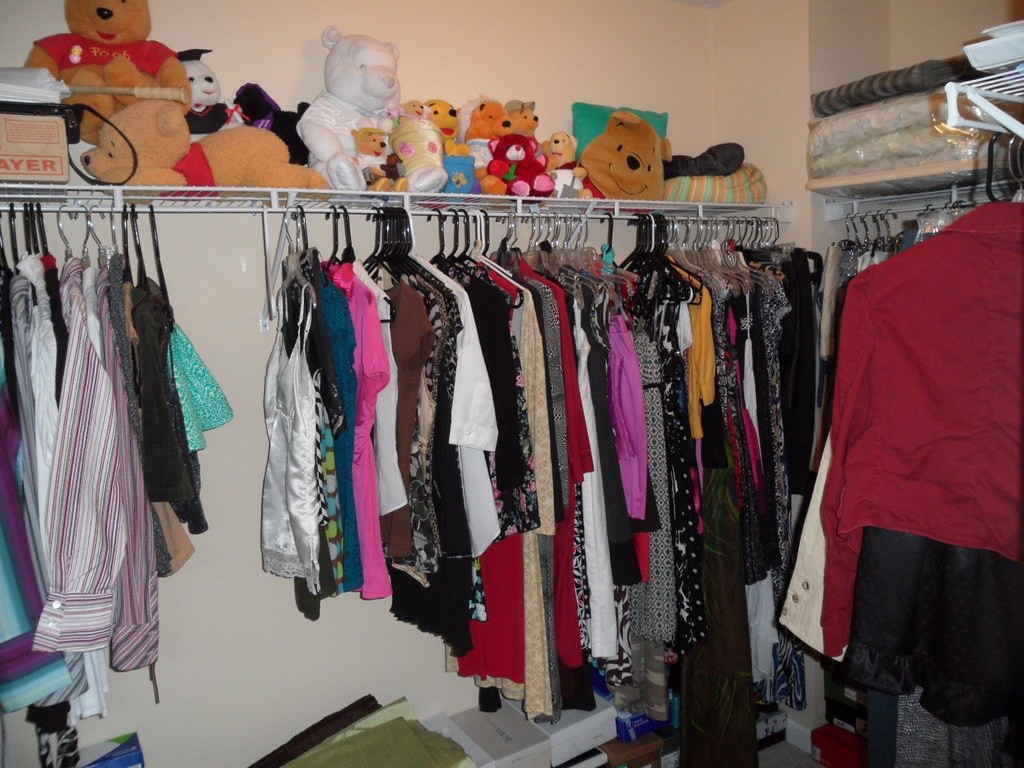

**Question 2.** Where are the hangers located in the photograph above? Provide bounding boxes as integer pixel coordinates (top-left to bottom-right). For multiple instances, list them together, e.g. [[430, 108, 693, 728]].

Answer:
[[0, 204, 784, 268], [836, 132, 1024, 247]]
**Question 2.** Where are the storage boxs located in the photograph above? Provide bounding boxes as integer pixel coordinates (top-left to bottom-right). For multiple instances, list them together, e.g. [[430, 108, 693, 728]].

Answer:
[[407, 689, 683, 768], [809, 666, 871, 768], [745, 708, 791, 755], [46, 731, 150, 768]]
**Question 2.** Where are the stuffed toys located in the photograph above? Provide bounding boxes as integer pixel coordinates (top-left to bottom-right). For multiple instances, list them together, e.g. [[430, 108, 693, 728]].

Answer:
[[24, 0, 587, 211], [579, 111, 673, 201]]
